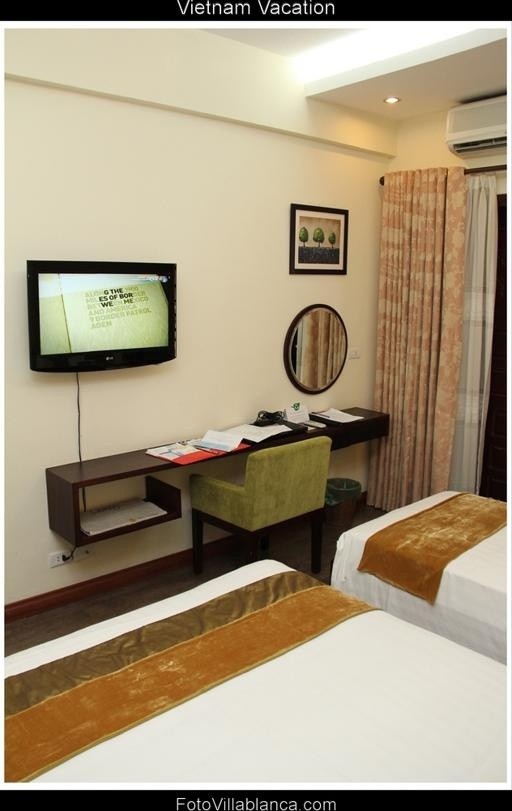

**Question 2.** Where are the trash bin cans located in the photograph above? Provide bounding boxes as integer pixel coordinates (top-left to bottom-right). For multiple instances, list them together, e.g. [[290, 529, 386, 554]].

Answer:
[[324, 478, 361, 527]]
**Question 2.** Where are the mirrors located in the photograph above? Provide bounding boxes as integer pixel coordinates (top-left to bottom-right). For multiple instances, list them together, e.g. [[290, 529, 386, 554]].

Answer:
[[283, 303, 349, 394]]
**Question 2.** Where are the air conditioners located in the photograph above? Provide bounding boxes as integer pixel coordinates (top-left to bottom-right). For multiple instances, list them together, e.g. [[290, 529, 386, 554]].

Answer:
[[445, 91, 507, 158]]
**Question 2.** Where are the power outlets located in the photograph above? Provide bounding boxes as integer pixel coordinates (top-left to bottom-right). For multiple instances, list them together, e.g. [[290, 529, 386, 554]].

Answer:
[[47, 551, 72, 569]]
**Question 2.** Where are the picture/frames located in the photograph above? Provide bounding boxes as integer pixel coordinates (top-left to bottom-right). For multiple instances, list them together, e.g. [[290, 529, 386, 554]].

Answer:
[[288, 202, 349, 275]]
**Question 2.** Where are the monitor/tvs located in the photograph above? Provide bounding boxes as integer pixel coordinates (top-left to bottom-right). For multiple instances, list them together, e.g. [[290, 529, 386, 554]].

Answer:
[[26, 260, 178, 373]]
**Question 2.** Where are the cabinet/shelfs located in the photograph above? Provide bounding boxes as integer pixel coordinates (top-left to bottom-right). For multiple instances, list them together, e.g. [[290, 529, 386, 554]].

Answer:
[[44, 405, 391, 546]]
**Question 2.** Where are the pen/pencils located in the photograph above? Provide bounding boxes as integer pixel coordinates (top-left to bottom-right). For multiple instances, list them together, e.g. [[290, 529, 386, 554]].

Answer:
[[196, 447, 218, 454], [311, 412, 330, 418], [168, 448, 184, 456]]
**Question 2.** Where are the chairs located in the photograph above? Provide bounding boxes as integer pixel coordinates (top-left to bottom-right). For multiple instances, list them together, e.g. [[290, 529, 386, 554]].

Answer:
[[189, 434, 333, 574]]
